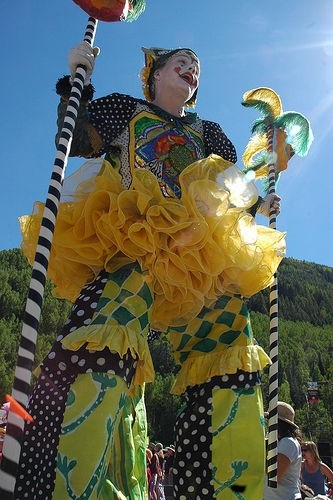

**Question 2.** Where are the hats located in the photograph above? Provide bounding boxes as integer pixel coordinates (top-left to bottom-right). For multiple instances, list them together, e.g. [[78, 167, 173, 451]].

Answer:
[[264, 401, 300, 430], [165, 444, 175, 452]]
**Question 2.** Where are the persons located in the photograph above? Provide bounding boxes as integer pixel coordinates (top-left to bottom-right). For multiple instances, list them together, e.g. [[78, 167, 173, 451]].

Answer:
[[0, 402, 333, 500], [14, 41, 281, 500]]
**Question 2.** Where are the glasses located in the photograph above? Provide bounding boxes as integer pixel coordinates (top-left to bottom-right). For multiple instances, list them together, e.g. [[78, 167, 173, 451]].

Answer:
[[302, 448, 309, 452]]
